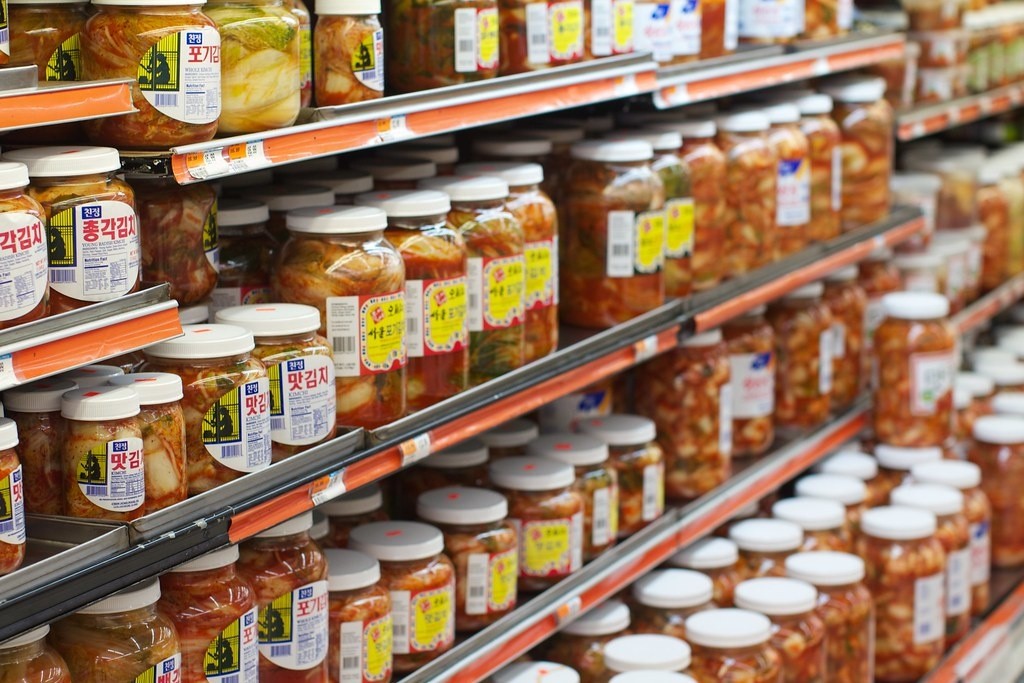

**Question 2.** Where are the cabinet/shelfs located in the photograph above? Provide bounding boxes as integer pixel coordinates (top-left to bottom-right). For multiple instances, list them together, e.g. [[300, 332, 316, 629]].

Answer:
[[0, 33, 1024, 683]]
